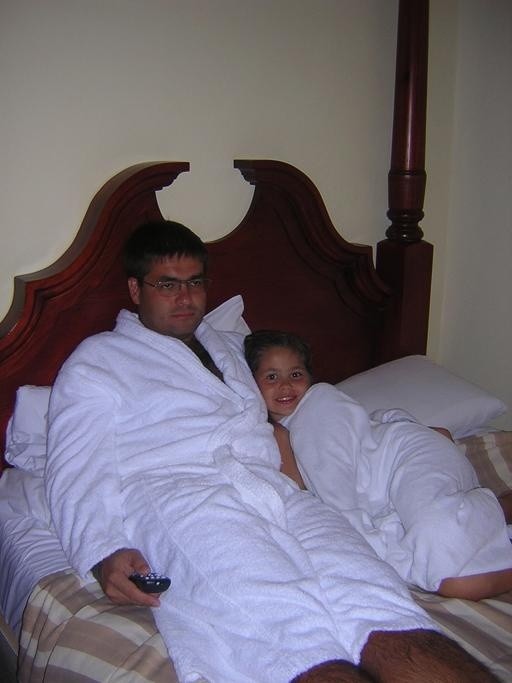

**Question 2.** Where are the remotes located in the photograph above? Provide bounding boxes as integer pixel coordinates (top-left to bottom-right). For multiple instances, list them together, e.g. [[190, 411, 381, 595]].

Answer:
[[130, 571, 171, 594]]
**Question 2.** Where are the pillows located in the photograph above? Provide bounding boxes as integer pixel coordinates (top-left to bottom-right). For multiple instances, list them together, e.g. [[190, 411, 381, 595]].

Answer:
[[330, 353, 508, 442], [1, 468, 58, 529]]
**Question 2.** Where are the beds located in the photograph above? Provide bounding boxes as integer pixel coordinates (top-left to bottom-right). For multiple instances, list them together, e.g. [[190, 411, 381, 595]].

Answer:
[[0, 156, 511, 682]]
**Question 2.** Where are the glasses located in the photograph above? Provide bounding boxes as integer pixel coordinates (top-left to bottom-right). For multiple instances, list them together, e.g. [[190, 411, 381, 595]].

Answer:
[[142, 278, 205, 297]]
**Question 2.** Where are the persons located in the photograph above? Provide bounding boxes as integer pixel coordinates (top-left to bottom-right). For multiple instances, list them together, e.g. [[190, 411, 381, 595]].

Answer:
[[245, 330, 512, 601], [45, 220, 500, 683]]
